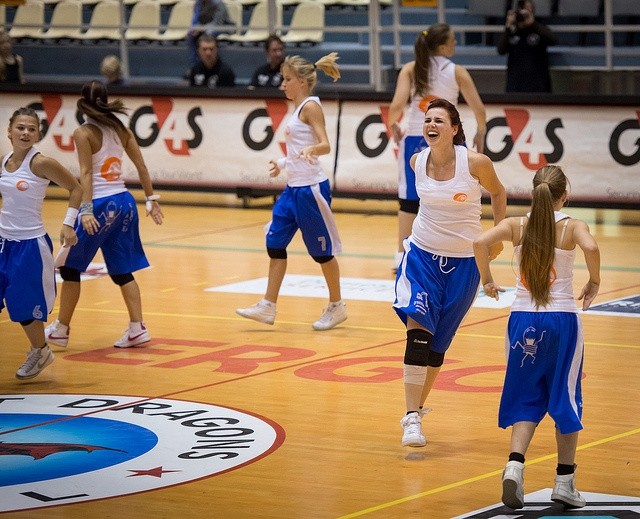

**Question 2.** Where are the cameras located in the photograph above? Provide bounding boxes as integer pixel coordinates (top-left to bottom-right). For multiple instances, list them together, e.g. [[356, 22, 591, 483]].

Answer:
[[513, 10, 524, 22]]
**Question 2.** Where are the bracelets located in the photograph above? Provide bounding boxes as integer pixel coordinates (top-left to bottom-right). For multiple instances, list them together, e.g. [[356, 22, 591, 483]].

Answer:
[[144, 194, 160, 201], [63, 207, 79, 228], [80, 202, 93, 215]]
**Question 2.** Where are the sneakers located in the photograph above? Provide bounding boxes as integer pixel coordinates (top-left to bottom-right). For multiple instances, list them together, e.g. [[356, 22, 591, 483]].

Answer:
[[312, 301, 348, 330], [45, 320, 70, 346], [113, 321, 151, 348], [551, 468, 586, 507], [402, 412, 427, 447], [236, 299, 276, 325], [501, 460, 524, 509], [15, 343, 55, 381]]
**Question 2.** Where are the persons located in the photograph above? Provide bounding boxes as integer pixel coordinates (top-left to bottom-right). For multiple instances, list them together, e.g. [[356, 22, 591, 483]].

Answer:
[[473, 166, 600, 509], [391, 99, 506, 450], [44, 79, 165, 347], [235, 51, 348, 331], [388, 23, 490, 269], [251, 35, 288, 88], [498, 0, 556, 93], [0, 107, 85, 380], [189, 35, 236, 87], [0, 26, 26, 83], [184, 0, 235, 79], [102, 56, 123, 86]]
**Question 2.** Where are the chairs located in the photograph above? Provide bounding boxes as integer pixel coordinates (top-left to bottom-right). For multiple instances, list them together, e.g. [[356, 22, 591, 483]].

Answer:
[[31, 0, 82, 44], [230, 2, 283, 48], [6, 0, 46, 43], [144, 2, 198, 47], [215, 0, 243, 46], [108, 1, 162, 45], [280, 1, 326, 48], [68, 0, 126, 44]]
